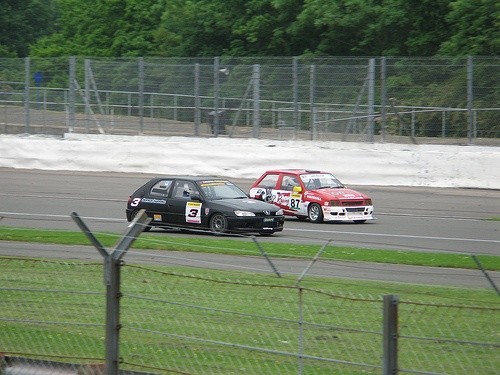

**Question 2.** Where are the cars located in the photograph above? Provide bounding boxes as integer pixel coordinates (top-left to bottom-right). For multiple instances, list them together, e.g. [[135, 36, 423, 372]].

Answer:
[[249, 170, 376, 225], [125, 175, 285, 235]]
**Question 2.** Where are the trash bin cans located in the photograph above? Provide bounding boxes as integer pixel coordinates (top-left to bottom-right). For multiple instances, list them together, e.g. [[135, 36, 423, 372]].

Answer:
[[208, 110, 225, 134]]
